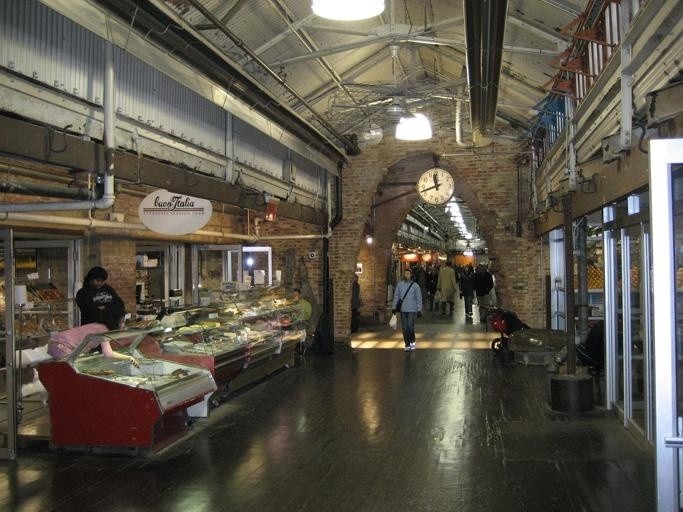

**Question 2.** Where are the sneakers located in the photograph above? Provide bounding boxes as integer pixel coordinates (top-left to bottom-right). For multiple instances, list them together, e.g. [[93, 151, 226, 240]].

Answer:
[[405, 346, 411, 352], [410, 342, 416, 350]]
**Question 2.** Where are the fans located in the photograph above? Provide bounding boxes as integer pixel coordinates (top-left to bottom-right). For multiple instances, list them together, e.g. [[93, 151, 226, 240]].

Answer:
[[328, 42, 445, 124]]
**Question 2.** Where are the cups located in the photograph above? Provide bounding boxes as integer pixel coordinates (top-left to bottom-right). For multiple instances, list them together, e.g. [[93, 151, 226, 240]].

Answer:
[[200, 297, 209, 306], [14, 285, 26, 304]]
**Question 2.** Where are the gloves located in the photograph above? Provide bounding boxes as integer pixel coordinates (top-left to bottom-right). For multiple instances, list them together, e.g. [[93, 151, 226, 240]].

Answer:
[[417, 311, 422, 318], [392, 309, 396, 315]]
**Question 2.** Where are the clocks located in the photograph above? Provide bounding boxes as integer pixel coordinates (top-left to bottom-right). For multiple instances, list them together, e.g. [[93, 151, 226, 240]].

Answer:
[[414, 153, 457, 206]]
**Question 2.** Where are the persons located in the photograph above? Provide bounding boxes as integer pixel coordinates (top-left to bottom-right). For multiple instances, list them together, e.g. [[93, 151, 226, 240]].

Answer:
[[351, 274, 363, 333], [585, 317, 622, 368], [46, 307, 142, 369], [391, 267, 423, 352], [411, 260, 494, 323], [288, 288, 313, 321], [75, 266, 127, 330]]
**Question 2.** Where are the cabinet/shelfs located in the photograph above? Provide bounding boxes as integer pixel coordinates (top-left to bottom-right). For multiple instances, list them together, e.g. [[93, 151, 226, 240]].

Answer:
[[571, 284, 682, 413]]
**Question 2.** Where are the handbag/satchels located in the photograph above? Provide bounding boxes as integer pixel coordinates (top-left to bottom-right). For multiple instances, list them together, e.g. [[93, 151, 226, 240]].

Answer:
[[396, 299, 403, 313]]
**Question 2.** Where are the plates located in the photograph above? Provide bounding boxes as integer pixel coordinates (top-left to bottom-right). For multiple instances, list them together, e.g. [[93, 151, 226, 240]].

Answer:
[[209, 333, 236, 340]]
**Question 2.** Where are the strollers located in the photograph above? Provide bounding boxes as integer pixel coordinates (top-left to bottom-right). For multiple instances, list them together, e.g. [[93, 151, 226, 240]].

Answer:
[[479, 303, 529, 354]]
